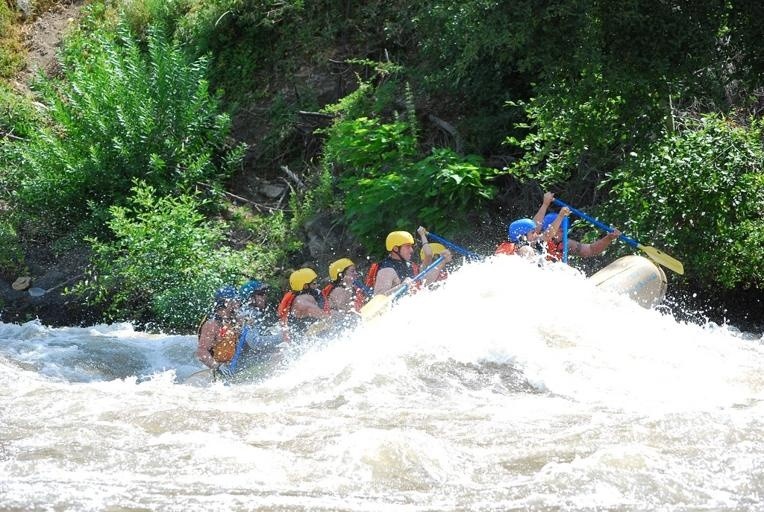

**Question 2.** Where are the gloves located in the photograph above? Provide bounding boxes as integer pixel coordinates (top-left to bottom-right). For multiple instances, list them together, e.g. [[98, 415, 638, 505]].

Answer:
[[177, 254, 668, 389]]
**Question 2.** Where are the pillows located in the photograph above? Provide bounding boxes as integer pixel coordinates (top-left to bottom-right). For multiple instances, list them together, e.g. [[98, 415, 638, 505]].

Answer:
[[385, 230, 415, 252], [540, 213, 570, 231], [289, 268, 317, 292], [213, 285, 239, 308], [509, 218, 537, 243], [328, 257, 354, 281], [419, 242, 447, 262], [239, 280, 268, 303]]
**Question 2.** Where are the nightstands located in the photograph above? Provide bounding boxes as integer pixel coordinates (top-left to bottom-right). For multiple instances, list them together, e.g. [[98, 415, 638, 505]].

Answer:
[[359, 254, 447, 323], [552, 198, 685, 274]]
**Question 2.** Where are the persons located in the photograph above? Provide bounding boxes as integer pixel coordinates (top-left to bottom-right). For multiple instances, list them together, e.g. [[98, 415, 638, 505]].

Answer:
[[365, 231, 422, 304], [197, 284, 243, 381], [417, 226, 453, 287], [322, 258, 365, 316], [229, 280, 283, 374], [274, 267, 328, 336], [495, 191, 626, 269]]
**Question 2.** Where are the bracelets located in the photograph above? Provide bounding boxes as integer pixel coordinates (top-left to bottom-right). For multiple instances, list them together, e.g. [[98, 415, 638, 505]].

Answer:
[[422, 242, 428, 245]]
